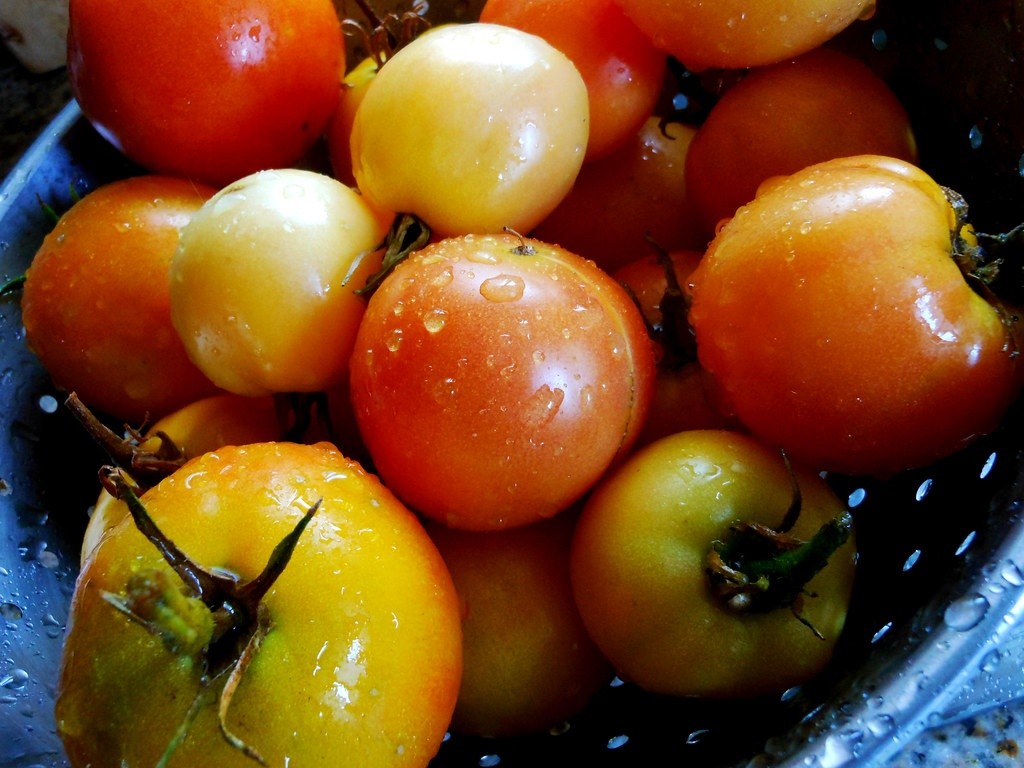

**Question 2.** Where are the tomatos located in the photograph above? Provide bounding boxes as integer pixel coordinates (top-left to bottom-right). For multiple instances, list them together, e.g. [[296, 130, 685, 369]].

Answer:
[[20, 0, 1024, 768]]
